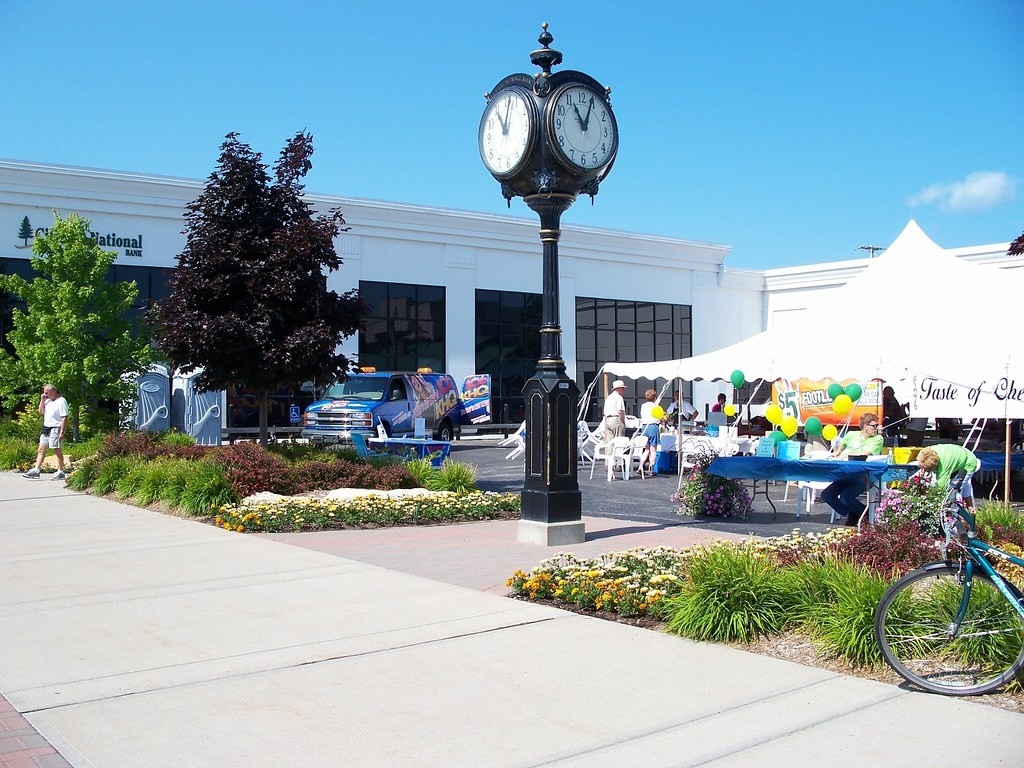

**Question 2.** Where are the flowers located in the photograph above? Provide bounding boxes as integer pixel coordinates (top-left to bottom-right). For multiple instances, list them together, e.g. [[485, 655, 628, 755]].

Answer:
[[670, 462, 756, 520]]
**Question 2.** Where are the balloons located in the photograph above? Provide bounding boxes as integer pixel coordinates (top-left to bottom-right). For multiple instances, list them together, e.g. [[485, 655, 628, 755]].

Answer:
[[805, 417, 837, 440], [766, 405, 798, 447], [651, 406, 664, 419], [828, 383, 862, 414], [731, 370, 745, 389], [724, 404, 735, 416]]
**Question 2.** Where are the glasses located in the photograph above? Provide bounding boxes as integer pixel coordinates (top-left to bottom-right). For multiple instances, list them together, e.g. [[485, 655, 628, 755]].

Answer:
[[867, 423, 880, 429]]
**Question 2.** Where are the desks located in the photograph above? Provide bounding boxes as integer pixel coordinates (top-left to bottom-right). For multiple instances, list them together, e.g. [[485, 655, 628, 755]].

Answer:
[[978, 451, 1023, 502], [366, 436, 451, 469], [704, 455, 921, 533], [656, 433, 751, 455]]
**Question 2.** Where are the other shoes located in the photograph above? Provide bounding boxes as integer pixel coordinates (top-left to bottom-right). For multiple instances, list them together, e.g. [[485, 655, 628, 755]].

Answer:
[[845, 512, 859, 526], [650, 473, 657, 477], [864, 508, 870, 520], [636, 470, 646, 476], [616, 468, 623, 472]]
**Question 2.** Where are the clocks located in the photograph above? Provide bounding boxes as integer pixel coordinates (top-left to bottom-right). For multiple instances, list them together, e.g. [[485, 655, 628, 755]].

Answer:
[[543, 81, 619, 175], [478, 84, 539, 179]]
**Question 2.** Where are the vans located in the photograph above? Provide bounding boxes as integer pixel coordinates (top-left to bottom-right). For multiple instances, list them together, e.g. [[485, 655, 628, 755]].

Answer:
[[300, 367, 492, 447]]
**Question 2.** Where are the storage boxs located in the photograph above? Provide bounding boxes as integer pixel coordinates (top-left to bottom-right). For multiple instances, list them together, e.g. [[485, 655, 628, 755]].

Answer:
[[883, 446, 922, 464]]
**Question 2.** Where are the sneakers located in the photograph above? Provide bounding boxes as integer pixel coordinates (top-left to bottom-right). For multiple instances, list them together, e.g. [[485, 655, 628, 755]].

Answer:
[[22, 469, 40, 479], [51, 471, 65, 481]]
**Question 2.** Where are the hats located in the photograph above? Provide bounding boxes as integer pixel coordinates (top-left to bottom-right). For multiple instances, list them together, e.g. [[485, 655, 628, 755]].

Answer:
[[612, 379, 628, 390]]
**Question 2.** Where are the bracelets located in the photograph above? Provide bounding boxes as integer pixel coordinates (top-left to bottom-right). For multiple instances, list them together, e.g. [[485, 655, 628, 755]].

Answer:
[[61, 431, 64, 433]]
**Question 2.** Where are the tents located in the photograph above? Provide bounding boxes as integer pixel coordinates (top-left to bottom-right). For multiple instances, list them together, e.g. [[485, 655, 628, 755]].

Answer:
[[603, 219, 1024, 510]]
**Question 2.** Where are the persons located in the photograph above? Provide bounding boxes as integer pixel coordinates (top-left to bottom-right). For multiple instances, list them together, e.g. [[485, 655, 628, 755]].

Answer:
[[667, 390, 699, 421], [604, 380, 627, 472], [712, 393, 726, 412], [935, 418, 962, 441], [917, 444, 978, 512], [22, 384, 69, 481], [821, 413, 884, 527], [636, 389, 661, 476], [883, 386, 928, 447]]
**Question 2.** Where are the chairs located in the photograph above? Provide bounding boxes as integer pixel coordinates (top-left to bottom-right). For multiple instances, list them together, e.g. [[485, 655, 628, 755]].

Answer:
[[496, 418, 527, 460], [351, 431, 391, 466], [782, 451, 882, 529], [577, 416, 649, 482]]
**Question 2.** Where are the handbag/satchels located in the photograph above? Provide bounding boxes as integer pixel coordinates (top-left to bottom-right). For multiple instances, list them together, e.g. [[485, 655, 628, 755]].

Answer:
[[41, 424, 56, 434]]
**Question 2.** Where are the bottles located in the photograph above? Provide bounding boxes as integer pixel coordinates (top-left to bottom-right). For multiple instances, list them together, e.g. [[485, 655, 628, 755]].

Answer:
[[670, 426, 675, 435], [894, 435, 898, 447], [1016, 444, 1020, 452]]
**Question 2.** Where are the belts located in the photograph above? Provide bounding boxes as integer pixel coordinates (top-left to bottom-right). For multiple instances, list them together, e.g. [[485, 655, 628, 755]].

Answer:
[[607, 415, 619, 417]]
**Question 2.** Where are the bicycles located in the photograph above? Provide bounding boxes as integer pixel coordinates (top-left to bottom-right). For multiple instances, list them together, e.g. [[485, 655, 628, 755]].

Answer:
[[874, 469, 1024, 697]]
